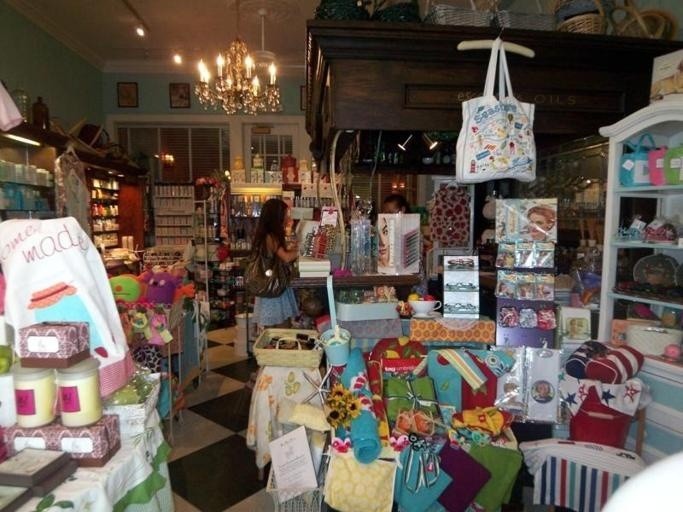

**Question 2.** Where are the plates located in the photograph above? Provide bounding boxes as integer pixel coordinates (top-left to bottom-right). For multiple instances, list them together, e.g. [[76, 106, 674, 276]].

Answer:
[[671, 261, 682, 293], [631, 252, 679, 289]]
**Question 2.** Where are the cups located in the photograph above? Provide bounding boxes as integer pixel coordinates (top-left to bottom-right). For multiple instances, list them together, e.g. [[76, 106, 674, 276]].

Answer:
[[410, 299, 442, 318], [348, 289, 363, 304], [318, 328, 352, 369], [338, 289, 348, 304], [0, 159, 49, 186]]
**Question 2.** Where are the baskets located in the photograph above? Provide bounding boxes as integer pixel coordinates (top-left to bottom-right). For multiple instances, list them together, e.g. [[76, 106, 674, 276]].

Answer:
[[252, 328, 324, 368], [424, 0, 677, 41]]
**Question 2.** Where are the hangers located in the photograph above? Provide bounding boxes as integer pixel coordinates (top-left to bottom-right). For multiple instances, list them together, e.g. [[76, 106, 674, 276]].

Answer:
[[455, 29, 538, 58]]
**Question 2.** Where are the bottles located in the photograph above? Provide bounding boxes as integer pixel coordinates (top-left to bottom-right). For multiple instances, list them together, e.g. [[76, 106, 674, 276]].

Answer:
[[295, 195, 331, 208], [0, 184, 43, 211], [10, 79, 31, 123], [573, 239, 586, 274], [33, 95, 49, 129], [232, 152, 317, 171], [584, 238, 600, 273]]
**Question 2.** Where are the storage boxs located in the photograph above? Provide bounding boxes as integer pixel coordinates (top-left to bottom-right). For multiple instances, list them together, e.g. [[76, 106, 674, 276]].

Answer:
[[5, 415, 122, 467]]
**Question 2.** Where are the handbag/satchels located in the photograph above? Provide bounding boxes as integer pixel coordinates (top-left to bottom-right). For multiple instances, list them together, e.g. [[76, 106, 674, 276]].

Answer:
[[619, 132, 655, 187], [663, 143, 682, 185], [454, 36, 535, 184], [243, 240, 289, 297], [648, 145, 666, 186]]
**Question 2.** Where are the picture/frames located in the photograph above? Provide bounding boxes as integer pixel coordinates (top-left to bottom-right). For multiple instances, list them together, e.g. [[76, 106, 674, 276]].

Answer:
[[116, 81, 139, 109], [168, 82, 191, 109]]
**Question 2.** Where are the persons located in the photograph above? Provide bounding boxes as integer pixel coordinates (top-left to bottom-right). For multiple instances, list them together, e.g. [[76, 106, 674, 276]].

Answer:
[[378, 217, 390, 267], [378, 196, 410, 213], [530, 382, 554, 403], [525, 204, 556, 241], [246, 198, 306, 336]]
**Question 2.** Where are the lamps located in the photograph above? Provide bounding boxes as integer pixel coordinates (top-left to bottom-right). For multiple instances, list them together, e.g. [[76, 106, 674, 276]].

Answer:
[[189, 1, 286, 118]]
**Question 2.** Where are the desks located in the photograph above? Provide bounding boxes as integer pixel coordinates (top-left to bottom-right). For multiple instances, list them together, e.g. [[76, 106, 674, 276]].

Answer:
[[1, 366, 180, 510]]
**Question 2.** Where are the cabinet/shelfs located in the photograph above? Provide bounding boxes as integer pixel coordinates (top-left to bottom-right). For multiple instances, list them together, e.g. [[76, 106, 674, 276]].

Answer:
[[0, 124, 425, 418], [589, 99, 681, 467]]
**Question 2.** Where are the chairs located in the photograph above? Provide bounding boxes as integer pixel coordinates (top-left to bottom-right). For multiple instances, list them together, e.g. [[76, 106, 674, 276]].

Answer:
[[430, 345, 523, 512], [539, 356, 648, 512]]
